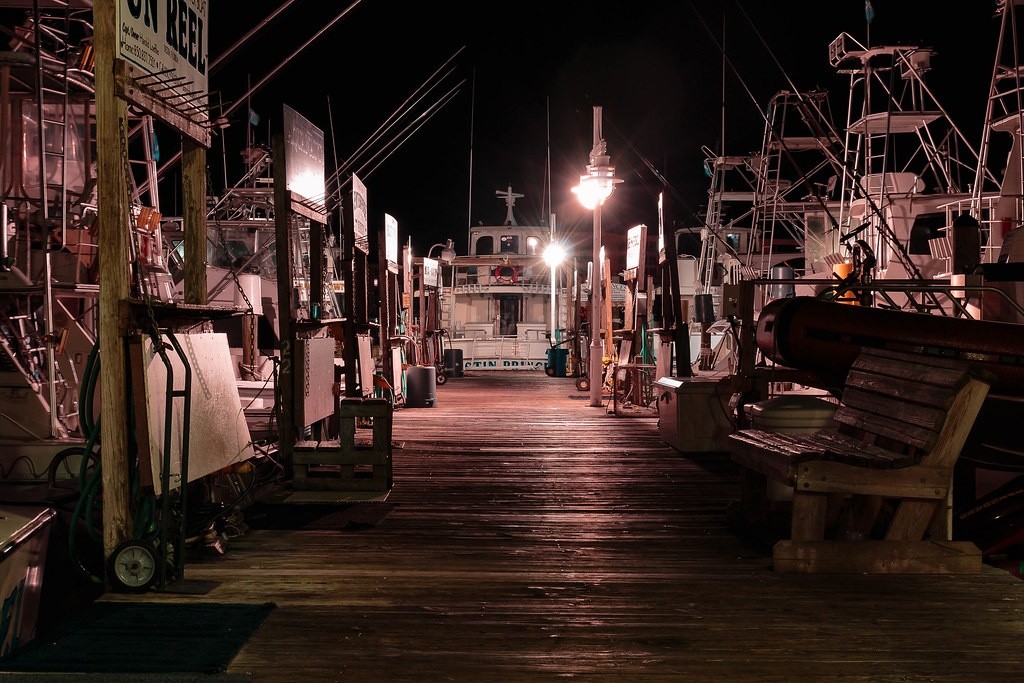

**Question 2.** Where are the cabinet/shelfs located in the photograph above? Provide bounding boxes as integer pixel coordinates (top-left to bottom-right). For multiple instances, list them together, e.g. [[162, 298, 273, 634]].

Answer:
[[653, 375, 731, 452]]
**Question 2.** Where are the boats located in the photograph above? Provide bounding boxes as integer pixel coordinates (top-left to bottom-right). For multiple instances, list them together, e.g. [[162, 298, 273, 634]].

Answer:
[[413, 96, 574, 372]]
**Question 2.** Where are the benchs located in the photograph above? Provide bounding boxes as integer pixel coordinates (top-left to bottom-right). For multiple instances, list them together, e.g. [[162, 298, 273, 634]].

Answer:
[[727, 345, 992, 574]]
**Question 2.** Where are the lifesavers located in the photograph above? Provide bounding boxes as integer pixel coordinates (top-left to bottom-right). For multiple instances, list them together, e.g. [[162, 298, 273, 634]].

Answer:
[[496, 265, 519, 284]]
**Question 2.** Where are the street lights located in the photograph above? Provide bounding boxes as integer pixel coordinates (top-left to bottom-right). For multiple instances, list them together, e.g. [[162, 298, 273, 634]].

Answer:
[[570, 105, 624, 408], [542, 213, 563, 345]]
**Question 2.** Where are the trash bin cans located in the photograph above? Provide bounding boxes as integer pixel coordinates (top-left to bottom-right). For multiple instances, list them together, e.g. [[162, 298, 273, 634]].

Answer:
[[545, 348, 570, 377], [742, 393, 846, 504]]
